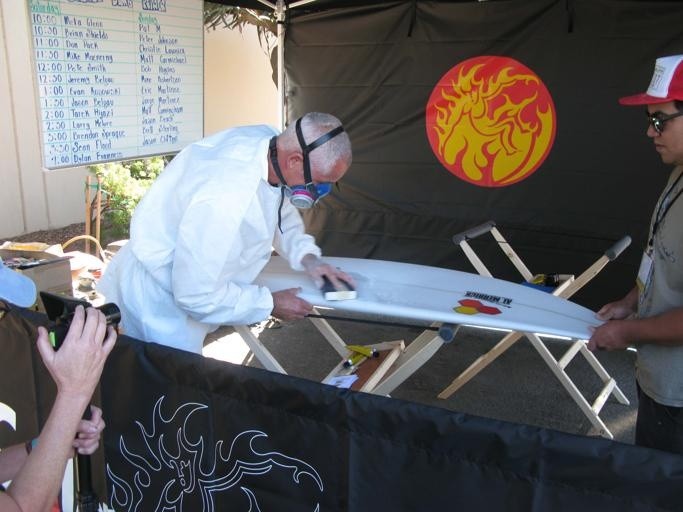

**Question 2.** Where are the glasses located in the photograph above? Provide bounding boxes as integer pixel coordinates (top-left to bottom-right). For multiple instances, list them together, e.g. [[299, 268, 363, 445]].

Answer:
[[647, 112, 683, 132]]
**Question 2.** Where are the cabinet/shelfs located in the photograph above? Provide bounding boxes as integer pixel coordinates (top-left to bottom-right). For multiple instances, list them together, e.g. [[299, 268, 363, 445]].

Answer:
[[0, 247, 77, 455]]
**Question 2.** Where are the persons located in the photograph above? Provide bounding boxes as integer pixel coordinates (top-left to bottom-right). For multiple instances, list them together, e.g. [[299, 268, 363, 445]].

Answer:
[[95, 111, 356, 355], [0, 256, 117, 511], [586, 55, 683, 454]]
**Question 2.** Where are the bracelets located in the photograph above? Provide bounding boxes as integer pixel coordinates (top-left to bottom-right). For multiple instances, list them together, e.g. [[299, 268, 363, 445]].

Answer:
[[24, 438, 36, 456]]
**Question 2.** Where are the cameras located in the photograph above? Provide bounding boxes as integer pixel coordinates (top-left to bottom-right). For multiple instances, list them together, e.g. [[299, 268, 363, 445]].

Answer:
[[39, 290, 121, 354]]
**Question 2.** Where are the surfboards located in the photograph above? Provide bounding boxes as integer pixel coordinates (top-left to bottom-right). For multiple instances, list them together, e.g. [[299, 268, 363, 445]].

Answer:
[[250, 255, 608, 341]]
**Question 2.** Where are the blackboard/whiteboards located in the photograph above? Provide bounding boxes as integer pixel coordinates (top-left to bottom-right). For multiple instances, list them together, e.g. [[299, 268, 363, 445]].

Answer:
[[24, 0, 205, 173]]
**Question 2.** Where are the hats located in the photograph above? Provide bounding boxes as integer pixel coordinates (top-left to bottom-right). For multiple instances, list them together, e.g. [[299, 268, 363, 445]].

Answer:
[[618, 55, 683, 106]]
